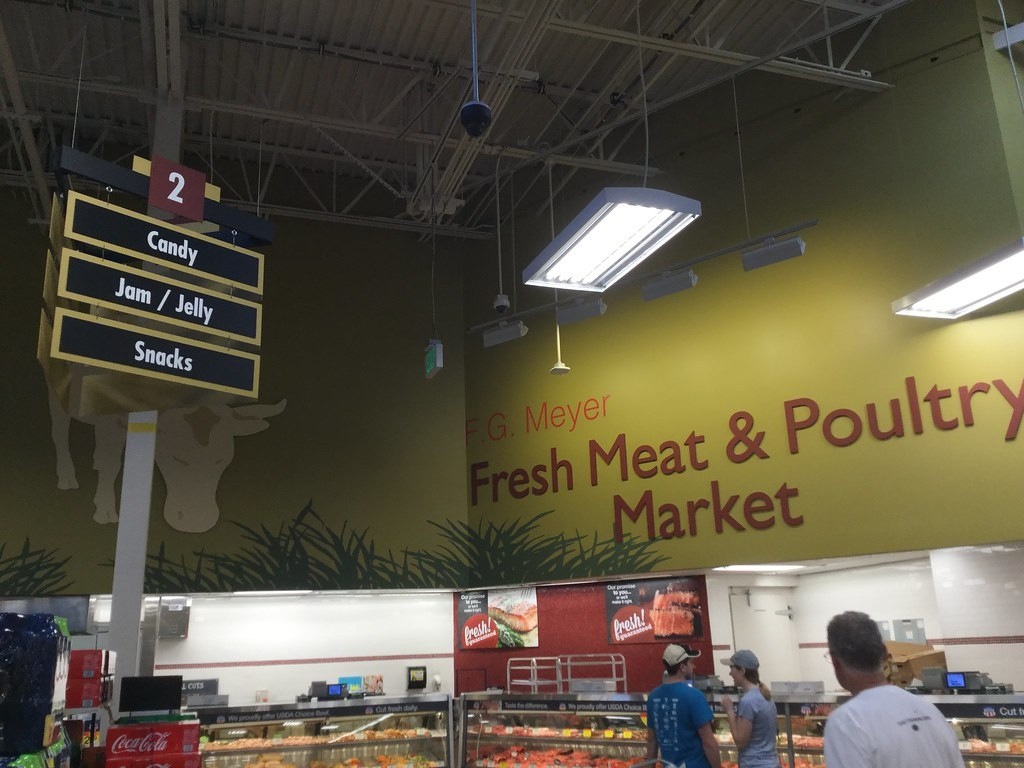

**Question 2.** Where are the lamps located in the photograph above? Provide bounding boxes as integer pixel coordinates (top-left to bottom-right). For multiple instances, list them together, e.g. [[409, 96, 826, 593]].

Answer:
[[482, 321, 529, 349], [521, 0, 704, 294], [554, 298, 608, 325], [641, 270, 699, 303], [740, 235, 808, 273], [889, 0, 1024, 324]]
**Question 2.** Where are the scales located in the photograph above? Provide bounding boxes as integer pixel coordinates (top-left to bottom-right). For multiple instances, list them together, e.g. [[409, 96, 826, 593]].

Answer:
[[312, 681, 349, 699], [922, 666, 996, 693]]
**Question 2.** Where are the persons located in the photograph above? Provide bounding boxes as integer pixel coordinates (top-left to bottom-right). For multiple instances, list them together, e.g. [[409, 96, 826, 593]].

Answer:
[[824, 611, 964, 768], [646, 642, 722, 768], [720, 649, 779, 768]]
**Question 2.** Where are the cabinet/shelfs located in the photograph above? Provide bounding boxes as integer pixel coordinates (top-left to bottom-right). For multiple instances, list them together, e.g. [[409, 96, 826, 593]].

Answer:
[[457, 689, 1024, 768], [506, 653, 628, 694], [181, 693, 454, 768]]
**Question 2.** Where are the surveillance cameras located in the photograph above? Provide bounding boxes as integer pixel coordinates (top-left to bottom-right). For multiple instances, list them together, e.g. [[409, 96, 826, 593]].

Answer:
[[492, 294, 510, 314], [458, 100, 493, 137]]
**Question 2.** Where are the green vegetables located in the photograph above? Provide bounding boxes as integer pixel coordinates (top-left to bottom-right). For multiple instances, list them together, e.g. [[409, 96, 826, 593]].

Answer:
[[495, 621, 524, 647]]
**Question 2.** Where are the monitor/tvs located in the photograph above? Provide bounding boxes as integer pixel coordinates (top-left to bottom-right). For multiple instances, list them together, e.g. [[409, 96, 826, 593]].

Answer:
[[945, 672, 966, 688], [328, 684, 342, 697]]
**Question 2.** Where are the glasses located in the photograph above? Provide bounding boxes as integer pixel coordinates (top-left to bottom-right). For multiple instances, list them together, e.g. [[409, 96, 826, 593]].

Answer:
[[824, 650, 836, 663]]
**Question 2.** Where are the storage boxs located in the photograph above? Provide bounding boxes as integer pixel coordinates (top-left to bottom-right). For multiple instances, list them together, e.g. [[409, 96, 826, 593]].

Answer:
[[65, 650, 117, 708], [104, 719, 203, 768], [882, 641, 948, 689]]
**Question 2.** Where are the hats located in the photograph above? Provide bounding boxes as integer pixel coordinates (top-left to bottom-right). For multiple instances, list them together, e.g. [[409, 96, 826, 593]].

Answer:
[[663, 643, 702, 669], [720, 649, 758, 670]]
